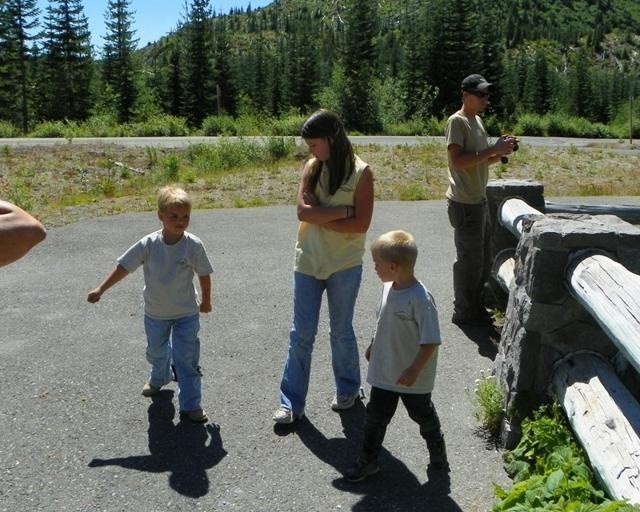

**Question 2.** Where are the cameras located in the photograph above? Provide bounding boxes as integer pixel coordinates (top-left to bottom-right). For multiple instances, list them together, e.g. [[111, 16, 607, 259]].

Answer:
[[505, 137, 520, 151]]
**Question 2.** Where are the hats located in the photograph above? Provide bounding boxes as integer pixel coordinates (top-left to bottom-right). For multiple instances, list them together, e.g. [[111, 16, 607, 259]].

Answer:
[[461, 74, 493, 92]]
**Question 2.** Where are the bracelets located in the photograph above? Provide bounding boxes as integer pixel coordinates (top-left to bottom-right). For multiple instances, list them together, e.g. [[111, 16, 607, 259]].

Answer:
[[345, 205, 349, 218]]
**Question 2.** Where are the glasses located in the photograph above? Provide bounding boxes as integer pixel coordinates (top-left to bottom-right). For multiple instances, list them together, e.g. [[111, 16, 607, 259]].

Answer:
[[469, 93, 490, 99]]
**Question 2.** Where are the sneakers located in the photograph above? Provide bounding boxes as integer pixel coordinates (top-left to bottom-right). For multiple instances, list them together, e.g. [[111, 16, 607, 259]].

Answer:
[[273, 406, 296, 423], [451, 311, 494, 326], [421, 480, 449, 497], [331, 392, 359, 409], [344, 461, 380, 481], [188, 408, 207, 421], [143, 383, 160, 395]]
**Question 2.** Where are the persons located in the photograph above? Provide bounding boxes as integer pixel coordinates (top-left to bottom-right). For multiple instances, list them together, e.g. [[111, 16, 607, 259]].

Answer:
[[271, 109, 374, 425], [445, 73, 521, 328], [87, 185, 214, 424], [0, 200, 49, 268], [342, 228, 453, 483]]
